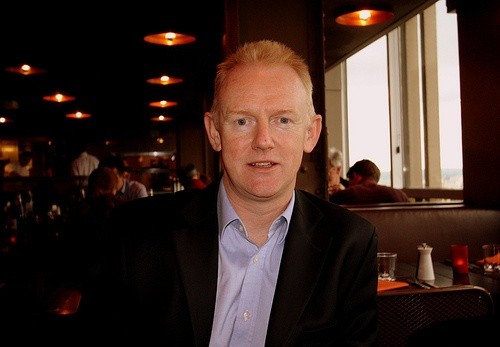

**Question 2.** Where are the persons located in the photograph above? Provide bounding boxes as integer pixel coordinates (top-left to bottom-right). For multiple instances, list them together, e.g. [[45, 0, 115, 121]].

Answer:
[[12, 144, 212, 244], [67, 39, 379, 347], [327, 148, 349, 196], [331, 160, 409, 205]]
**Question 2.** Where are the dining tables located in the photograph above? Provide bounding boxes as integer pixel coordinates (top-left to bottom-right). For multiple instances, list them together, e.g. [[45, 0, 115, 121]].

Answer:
[[377, 262, 500, 296]]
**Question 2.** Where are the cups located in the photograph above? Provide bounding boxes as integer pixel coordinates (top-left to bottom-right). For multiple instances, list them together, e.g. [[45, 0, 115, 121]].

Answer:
[[377, 252, 397, 281], [451, 244, 469, 275], [417, 246, 435, 280], [482, 244, 500, 274]]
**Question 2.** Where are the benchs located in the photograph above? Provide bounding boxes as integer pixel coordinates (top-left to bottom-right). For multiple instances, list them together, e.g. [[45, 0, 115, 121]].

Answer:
[[348, 210, 500, 262]]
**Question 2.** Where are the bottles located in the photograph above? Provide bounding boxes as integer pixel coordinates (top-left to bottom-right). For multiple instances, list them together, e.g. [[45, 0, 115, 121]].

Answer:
[[7, 190, 33, 231], [173, 177, 185, 193]]
